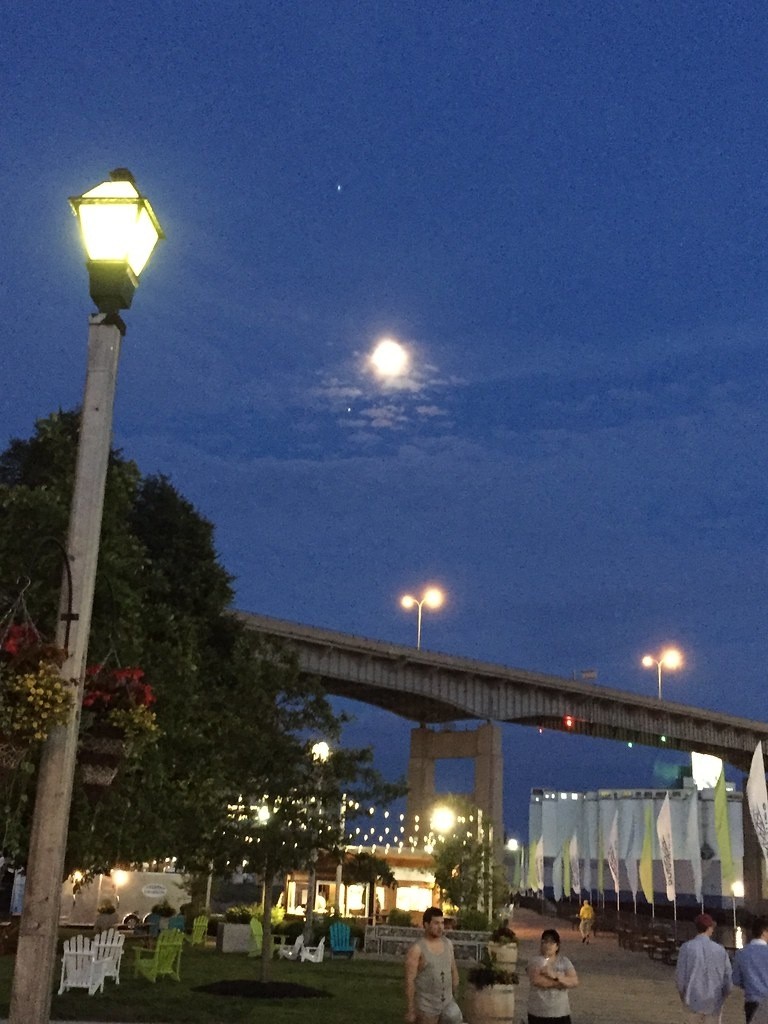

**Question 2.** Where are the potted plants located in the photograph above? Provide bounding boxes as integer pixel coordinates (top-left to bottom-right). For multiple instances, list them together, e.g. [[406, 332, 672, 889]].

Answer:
[[463, 945, 520, 1020], [487, 918, 517, 973]]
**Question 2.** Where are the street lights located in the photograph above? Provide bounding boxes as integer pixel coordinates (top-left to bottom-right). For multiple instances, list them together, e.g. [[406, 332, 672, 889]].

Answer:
[[258, 806, 280, 906], [642, 644, 684, 701], [431, 809, 455, 914], [400, 587, 445, 650], [507, 839, 525, 889], [0, 168, 163, 1024], [302, 741, 333, 947]]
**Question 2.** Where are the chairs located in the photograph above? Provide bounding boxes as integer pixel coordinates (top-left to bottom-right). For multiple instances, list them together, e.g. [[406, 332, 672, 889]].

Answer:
[[246, 917, 285, 958], [143, 913, 161, 938], [181, 915, 209, 947], [364, 925, 383, 957], [91, 927, 126, 984], [300, 936, 325, 963], [58, 936, 106, 995], [279, 933, 304, 961], [132, 927, 184, 984], [329, 921, 355, 961], [168, 915, 186, 932]]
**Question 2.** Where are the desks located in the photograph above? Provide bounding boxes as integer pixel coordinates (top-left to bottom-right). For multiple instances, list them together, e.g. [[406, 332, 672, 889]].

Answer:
[[112, 930, 155, 949]]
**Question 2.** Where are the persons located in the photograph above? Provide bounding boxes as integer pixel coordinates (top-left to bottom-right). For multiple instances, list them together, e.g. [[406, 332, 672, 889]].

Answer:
[[674, 915, 768, 1024], [579, 900, 593, 946], [525, 929, 578, 1024], [404, 907, 463, 1024]]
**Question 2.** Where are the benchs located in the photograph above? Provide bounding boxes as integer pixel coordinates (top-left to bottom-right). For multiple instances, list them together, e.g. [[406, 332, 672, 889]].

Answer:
[[375, 925, 493, 957]]
